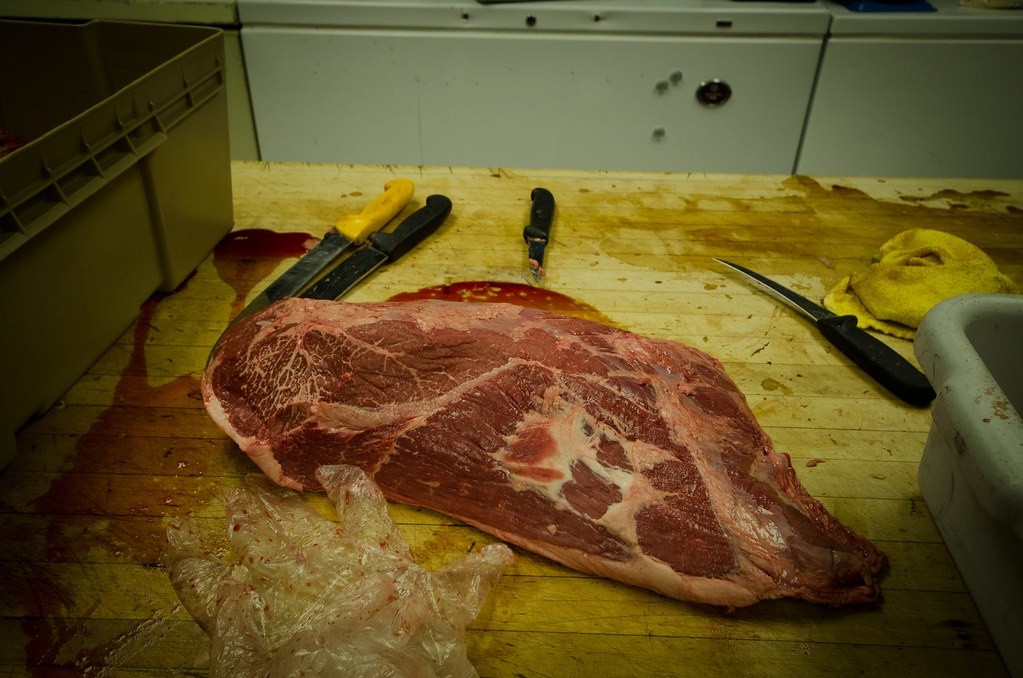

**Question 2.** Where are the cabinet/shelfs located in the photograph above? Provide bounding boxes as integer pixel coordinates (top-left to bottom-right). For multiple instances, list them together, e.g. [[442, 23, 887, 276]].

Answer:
[[0, 0, 1023, 182]]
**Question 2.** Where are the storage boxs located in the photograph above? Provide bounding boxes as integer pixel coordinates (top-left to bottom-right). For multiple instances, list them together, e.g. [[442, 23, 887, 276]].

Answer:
[[0, 19, 236, 471], [912, 293, 1022, 678]]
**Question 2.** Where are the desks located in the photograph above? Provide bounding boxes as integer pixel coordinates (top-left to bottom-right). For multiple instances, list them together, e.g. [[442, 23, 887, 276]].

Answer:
[[0, 164, 1023, 678]]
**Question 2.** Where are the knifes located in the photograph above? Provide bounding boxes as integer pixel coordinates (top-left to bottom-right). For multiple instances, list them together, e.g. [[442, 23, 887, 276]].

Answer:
[[206, 178, 415, 369], [523, 188, 554, 284], [301, 195, 452, 301], [713, 257, 936, 407]]
